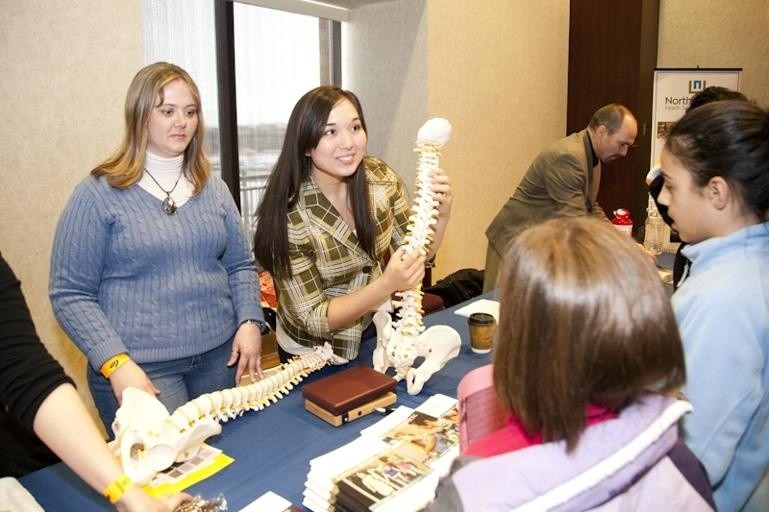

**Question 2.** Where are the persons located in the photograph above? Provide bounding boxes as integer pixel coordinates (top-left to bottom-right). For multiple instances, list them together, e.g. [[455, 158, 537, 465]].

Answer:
[[422, 219, 723, 511], [47, 62, 266, 443], [250, 87, 454, 368], [644, 85, 749, 293], [656, 100, 768, 511], [0, 253, 195, 512], [480, 102, 638, 295]]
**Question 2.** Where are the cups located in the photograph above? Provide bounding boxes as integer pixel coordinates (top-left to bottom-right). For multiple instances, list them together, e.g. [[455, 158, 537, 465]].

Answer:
[[467, 313, 497, 354]]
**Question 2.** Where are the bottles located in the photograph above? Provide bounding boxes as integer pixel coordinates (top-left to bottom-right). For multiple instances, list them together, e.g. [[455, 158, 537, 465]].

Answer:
[[643, 206, 666, 256]]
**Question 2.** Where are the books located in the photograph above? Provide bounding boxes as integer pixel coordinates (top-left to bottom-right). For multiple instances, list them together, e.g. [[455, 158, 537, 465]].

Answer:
[[302, 392, 467, 512]]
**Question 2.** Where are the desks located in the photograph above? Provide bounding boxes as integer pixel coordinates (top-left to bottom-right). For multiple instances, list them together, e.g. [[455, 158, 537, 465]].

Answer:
[[17, 251, 677, 512]]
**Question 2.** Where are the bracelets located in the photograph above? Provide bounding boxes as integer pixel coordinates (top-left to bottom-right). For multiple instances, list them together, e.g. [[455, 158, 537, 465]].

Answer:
[[247, 319, 263, 328], [101, 352, 130, 379], [102, 474, 136, 505]]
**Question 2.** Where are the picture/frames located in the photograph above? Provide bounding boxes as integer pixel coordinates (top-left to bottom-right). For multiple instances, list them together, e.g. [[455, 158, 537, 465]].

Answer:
[[649, 68, 743, 169]]
